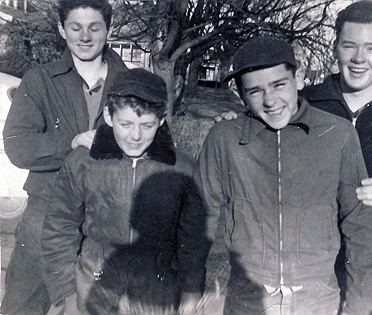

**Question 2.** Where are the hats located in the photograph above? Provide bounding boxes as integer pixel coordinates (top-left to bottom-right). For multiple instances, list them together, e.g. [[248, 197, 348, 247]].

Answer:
[[221, 36, 296, 84], [107, 68, 167, 104]]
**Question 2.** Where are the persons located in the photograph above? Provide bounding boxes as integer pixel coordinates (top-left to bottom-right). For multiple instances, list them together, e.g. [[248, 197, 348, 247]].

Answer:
[[0, 0, 131, 315], [39, 68, 207, 315], [208, 0, 372, 207], [176, 36, 372, 315]]
